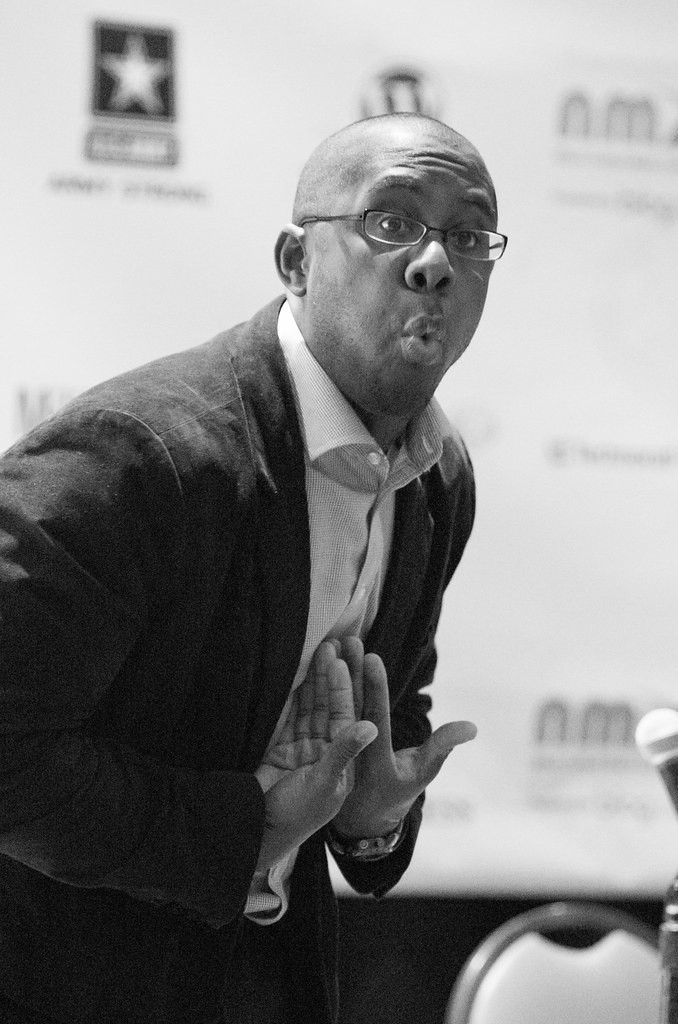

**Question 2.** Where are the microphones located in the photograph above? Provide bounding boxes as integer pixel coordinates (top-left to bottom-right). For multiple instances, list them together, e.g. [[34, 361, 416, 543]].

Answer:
[[636, 707, 678, 812]]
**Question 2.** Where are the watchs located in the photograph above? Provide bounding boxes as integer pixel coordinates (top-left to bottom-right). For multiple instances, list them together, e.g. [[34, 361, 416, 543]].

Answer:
[[327, 819, 404, 862]]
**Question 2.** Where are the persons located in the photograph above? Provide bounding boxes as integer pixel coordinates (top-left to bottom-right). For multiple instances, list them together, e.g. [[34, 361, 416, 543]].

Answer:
[[0, 110, 507, 1024]]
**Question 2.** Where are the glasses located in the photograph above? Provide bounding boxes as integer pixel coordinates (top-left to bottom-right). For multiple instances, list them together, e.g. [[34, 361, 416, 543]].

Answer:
[[299, 208, 508, 262]]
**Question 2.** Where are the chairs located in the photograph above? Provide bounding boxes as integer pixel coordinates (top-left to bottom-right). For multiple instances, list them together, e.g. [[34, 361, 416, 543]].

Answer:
[[447, 903, 665, 1024]]
